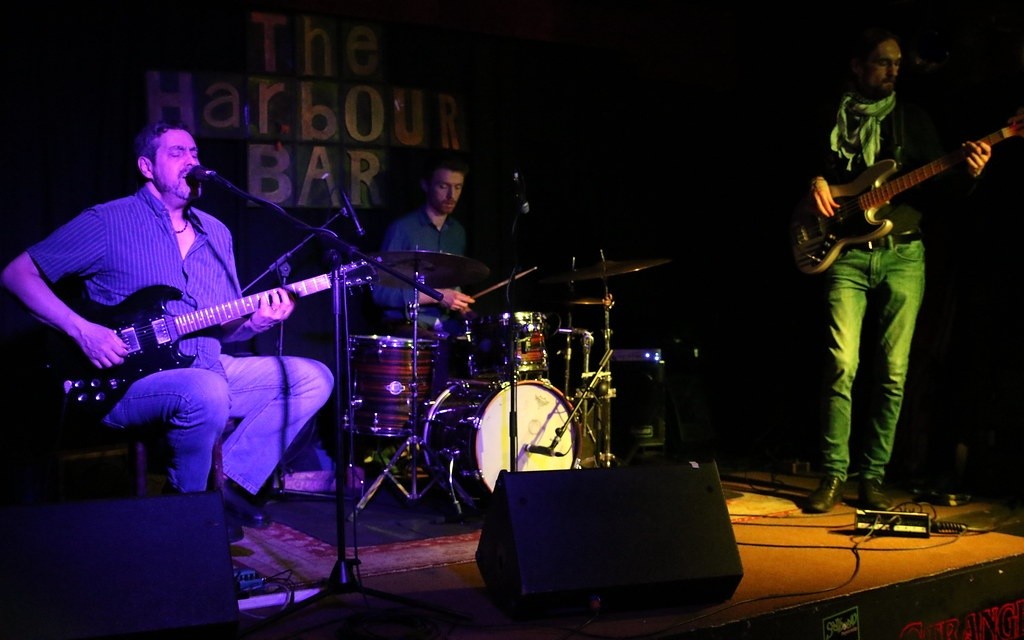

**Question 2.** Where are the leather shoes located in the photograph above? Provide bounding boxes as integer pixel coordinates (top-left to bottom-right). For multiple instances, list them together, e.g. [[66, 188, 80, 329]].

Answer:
[[804, 476, 843, 513], [228, 484, 270, 526], [225, 516, 244, 542], [858, 490, 891, 510]]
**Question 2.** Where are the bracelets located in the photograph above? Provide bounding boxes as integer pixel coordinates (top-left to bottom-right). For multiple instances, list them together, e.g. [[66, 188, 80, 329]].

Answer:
[[817, 177, 824, 180]]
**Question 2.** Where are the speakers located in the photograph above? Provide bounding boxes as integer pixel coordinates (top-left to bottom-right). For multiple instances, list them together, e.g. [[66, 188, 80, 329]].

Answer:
[[0, 489, 242, 640], [475, 459, 746, 624], [608, 361, 666, 450]]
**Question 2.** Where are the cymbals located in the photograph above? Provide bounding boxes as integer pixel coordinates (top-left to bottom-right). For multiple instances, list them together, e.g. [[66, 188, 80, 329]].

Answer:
[[362, 249, 490, 290], [563, 295, 605, 307], [539, 257, 673, 284]]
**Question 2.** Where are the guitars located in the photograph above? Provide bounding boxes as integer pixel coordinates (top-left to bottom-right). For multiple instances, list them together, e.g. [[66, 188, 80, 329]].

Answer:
[[15, 256, 382, 432], [790, 109, 1023, 275]]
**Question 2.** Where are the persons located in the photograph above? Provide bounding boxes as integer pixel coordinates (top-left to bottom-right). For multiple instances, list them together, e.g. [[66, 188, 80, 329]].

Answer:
[[0, 120, 335, 545], [369, 154, 511, 467], [787, 30, 993, 513]]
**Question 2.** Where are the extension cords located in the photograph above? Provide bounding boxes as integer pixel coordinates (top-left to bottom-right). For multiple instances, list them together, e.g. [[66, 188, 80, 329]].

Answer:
[[854, 509, 933, 536]]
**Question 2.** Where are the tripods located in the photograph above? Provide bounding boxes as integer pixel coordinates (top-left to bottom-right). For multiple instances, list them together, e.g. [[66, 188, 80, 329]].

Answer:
[[216, 176, 481, 640]]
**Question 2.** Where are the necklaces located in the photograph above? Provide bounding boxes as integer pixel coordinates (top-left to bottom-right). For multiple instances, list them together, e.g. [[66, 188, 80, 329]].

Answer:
[[175, 219, 188, 233]]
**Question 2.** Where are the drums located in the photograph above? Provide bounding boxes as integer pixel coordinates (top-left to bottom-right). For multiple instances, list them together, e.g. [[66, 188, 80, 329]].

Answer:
[[468, 310, 550, 380], [421, 377, 583, 518], [344, 333, 435, 438]]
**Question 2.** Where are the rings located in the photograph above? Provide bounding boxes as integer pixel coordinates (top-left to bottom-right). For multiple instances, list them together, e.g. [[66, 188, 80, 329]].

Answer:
[[272, 307, 277, 311]]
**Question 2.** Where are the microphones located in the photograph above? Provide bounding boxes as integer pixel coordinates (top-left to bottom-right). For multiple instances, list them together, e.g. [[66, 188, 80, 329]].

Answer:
[[337, 185, 366, 238], [512, 172, 530, 214], [524, 444, 565, 457], [189, 166, 217, 183]]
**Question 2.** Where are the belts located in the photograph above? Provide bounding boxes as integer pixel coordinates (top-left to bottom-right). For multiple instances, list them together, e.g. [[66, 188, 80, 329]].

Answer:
[[850, 231, 921, 249]]
